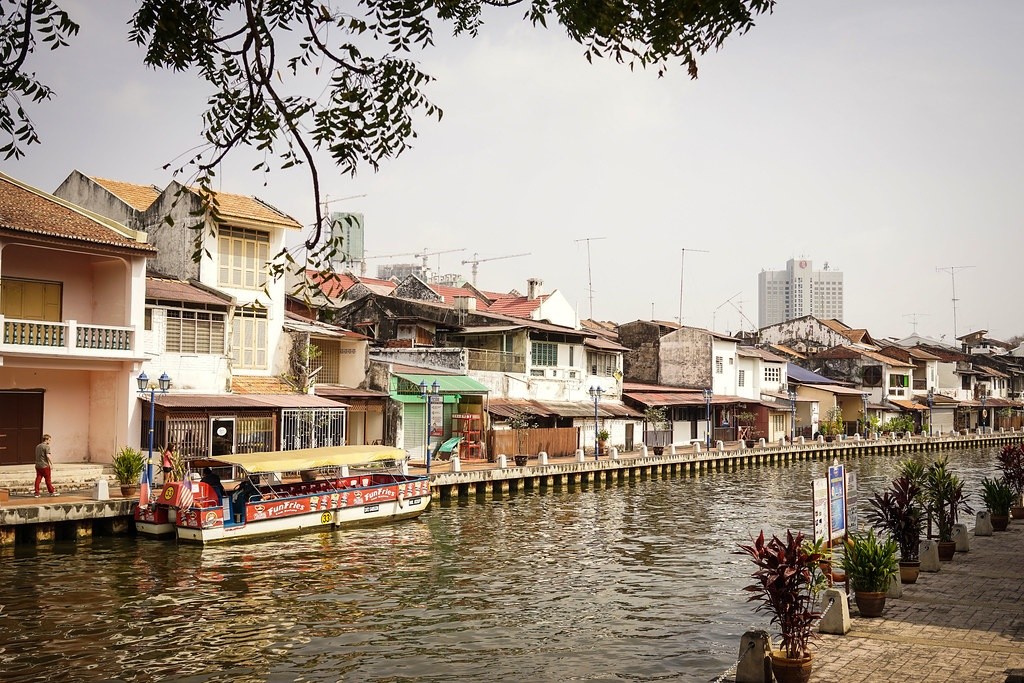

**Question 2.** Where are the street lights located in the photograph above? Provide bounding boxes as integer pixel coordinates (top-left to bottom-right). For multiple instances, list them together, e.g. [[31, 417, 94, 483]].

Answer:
[[861, 394, 870, 440], [589, 385, 602, 461], [788, 390, 798, 447], [419, 377, 440, 474], [979, 395, 987, 435], [702, 388, 713, 451], [136, 370, 171, 492], [927, 390, 935, 437]]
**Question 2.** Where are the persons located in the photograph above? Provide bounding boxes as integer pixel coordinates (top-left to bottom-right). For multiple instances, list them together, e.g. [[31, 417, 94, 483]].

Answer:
[[199, 467, 224, 507], [163, 444, 177, 487], [33, 434, 60, 498]]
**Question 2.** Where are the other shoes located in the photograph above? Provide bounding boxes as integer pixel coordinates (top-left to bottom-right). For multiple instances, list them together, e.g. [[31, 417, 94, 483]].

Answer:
[[35, 494, 41, 498], [50, 492, 60, 497]]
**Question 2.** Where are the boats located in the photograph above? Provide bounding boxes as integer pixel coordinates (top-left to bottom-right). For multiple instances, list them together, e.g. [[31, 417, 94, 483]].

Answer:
[[133, 445, 433, 547]]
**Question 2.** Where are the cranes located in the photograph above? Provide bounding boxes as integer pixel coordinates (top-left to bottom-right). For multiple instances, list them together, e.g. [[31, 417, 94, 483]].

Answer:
[[352, 248, 419, 278], [415, 247, 469, 269], [461, 250, 532, 288], [320, 192, 368, 271]]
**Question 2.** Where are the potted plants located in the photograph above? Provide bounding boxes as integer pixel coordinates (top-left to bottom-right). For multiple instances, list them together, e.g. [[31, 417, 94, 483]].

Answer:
[[295, 406, 331, 482], [826, 406, 842, 442], [738, 412, 758, 448], [895, 453, 965, 561], [111, 445, 147, 498], [837, 524, 902, 618], [857, 416, 913, 440], [599, 431, 608, 454], [863, 473, 926, 584], [730, 530, 832, 683], [979, 476, 1016, 532], [996, 443, 1024, 519], [636, 405, 672, 455], [505, 407, 539, 466]]
